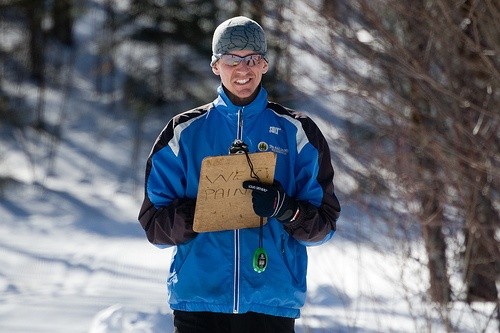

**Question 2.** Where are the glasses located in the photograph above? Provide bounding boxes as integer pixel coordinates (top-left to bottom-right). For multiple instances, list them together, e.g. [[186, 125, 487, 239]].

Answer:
[[213, 53, 265, 67]]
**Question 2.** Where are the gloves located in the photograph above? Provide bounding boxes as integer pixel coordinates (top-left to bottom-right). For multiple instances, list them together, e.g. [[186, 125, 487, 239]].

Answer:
[[243, 179, 290, 218]]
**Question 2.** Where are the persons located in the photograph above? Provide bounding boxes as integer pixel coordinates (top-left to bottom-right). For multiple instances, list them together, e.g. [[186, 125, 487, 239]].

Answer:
[[138, 16, 341, 333]]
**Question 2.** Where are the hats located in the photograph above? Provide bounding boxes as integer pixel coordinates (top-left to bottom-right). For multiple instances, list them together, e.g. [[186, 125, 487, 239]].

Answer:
[[210, 16, 268, 67]]
